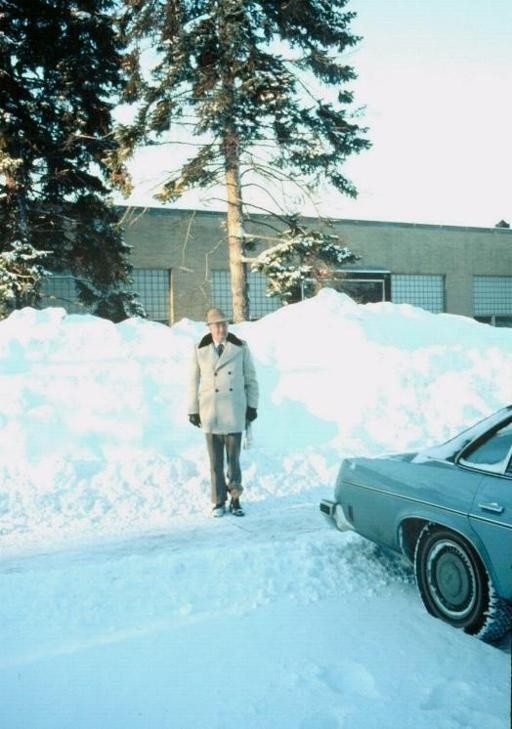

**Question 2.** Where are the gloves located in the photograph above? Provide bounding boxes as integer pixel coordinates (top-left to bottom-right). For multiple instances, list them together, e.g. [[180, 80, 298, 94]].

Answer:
[[189, 414, 201, 428], [245, 407, 257, 421]]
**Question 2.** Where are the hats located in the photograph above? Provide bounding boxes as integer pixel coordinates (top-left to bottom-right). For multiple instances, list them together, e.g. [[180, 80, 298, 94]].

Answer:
[[205, 308, 230, 325]]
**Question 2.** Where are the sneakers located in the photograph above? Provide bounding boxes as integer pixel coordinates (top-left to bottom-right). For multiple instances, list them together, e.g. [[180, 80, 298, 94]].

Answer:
[[212, 499, 244, 517]]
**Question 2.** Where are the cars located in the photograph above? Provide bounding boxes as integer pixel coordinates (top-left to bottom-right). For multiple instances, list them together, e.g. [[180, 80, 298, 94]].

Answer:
[[321, 403, 512, 651]]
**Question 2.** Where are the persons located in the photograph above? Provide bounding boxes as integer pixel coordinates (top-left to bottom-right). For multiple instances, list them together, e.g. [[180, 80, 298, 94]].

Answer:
[[185, 306, 261, 518]]
